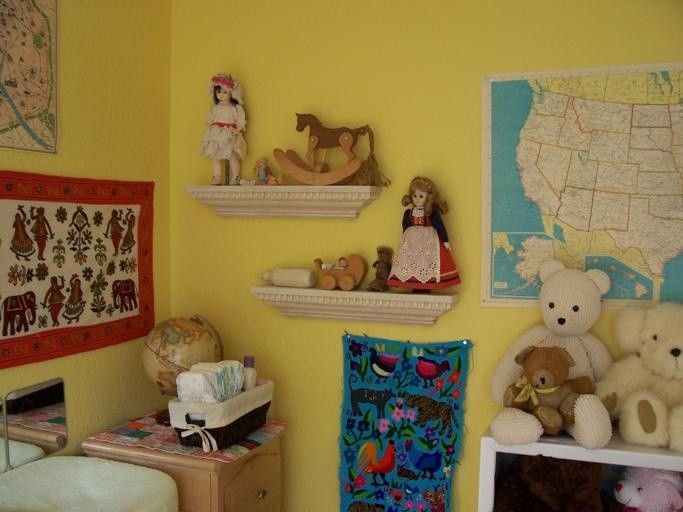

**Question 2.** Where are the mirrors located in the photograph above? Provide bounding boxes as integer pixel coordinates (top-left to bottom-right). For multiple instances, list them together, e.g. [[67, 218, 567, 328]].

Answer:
[[0, 378, 70, 475]]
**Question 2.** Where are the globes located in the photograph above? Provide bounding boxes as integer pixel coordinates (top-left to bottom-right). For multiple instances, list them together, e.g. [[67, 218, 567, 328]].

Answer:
[[142, 314, 224, 426]]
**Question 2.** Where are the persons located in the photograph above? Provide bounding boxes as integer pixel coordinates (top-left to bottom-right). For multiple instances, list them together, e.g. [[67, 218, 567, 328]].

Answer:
[[200, 72, 463, 295]]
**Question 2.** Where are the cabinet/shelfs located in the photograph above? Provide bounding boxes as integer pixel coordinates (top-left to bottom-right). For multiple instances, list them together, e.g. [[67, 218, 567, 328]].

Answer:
[[187, 178, 462, 324], [477, 426, 683, 512], [80, 420, 285, 512]]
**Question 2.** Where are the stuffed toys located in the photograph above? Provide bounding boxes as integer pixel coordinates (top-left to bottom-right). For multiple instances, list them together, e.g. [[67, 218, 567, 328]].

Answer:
[[487, 258, 683, 511]]
